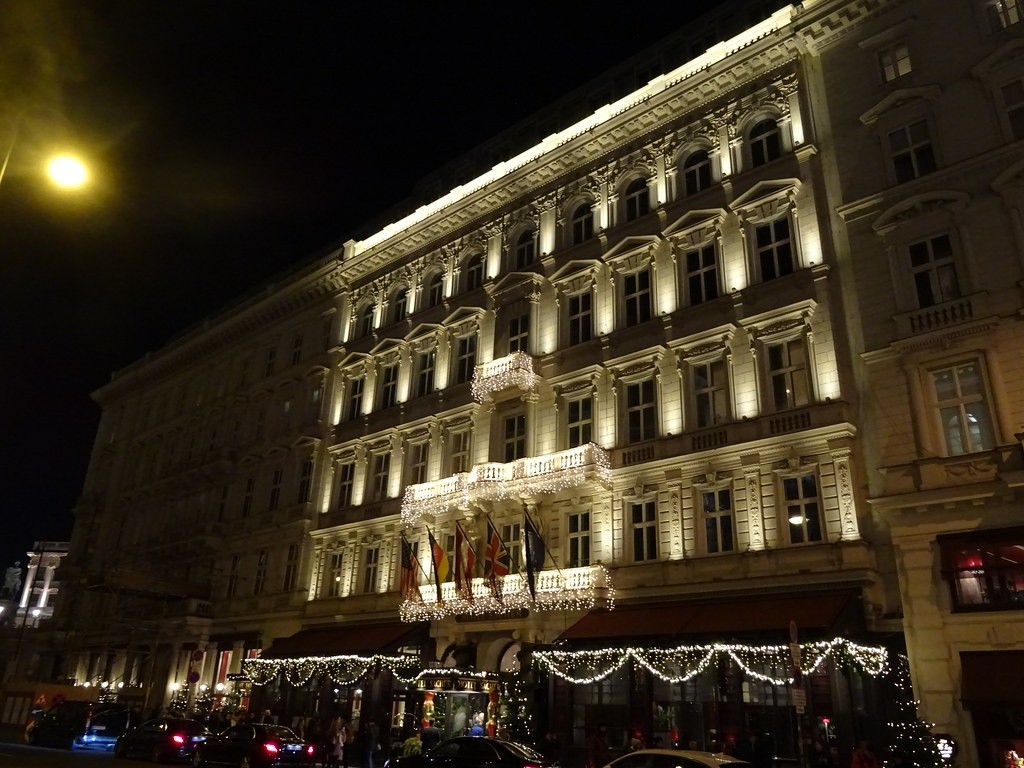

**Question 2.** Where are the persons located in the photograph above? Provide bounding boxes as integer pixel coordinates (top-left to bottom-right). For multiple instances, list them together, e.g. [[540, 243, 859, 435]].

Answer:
[[850, 745, 880, 768], [197, 703, 380, 768], [404, 709, 484, 758], [540, 723, 697, 768]]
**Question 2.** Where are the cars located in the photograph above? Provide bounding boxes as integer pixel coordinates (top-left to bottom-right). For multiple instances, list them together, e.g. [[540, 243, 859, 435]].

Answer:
[[113, 716, 215, 765], [383, 736, 560, 768], [191, 722, 317, 768], [600, 748, 751, 768], [23, 699, 136, 753]]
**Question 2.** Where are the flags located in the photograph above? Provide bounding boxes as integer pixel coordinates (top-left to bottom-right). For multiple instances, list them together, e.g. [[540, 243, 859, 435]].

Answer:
[[454, 521, 476, 606], [524, 508, 545, 602], [427, 527, 449, 607], [483, 515, 509, 605], [400, 535, 419, 603]]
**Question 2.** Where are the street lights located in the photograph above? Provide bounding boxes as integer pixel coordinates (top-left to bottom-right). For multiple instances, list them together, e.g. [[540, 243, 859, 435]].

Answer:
[[0, 104, 95, 197]]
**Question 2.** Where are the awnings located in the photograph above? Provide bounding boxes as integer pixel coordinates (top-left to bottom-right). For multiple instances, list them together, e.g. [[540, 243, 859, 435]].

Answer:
[[441, 638, 521, 673], [257, 622, 430, 660], [936, 527, 1024, 579], [551, 587, 860, 644]]
